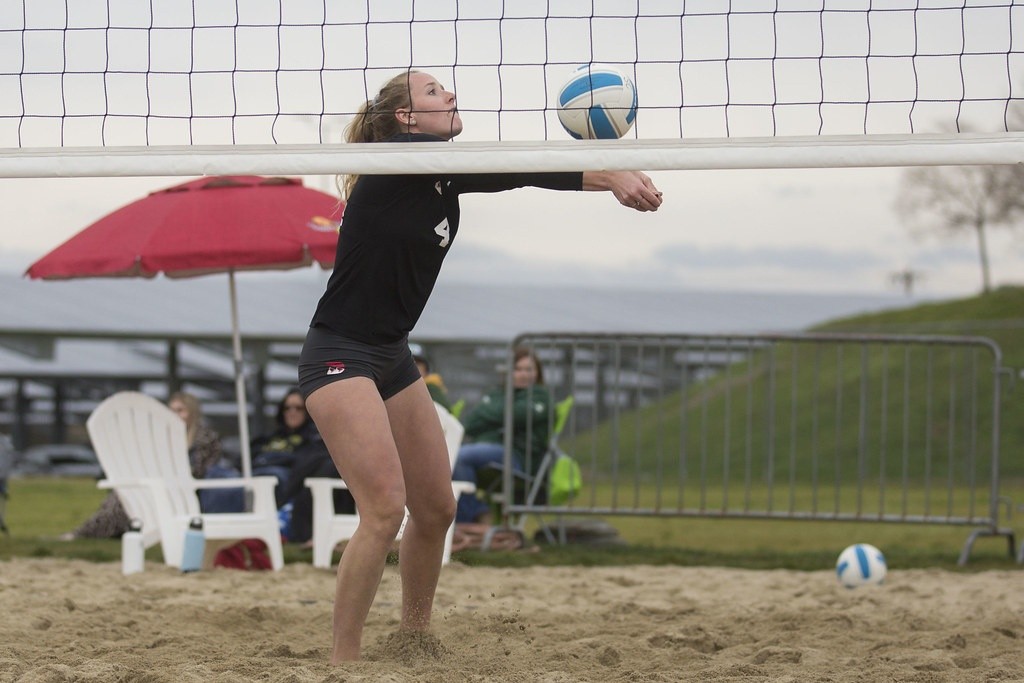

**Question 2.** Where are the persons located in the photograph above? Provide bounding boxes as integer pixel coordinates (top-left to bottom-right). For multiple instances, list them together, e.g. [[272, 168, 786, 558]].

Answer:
[[166, 392, 221, 479], [408, 342, 452, 411], [452, 349, 553, 526], [296, 71, 664, 668], [209, 387, 320, 539]]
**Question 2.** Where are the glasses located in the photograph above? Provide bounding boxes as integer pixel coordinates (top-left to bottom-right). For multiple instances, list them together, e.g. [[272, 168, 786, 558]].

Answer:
[[282, 405, 305, 412]]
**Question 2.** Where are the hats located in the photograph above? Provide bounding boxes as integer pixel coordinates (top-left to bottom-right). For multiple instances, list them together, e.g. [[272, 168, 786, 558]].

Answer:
[[408, 343, 430, 373]]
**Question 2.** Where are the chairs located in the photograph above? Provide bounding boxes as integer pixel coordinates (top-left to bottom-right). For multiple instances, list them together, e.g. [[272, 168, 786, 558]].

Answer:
[[87, 391, 285, 574], [303, 403, 476, 570], [483, 396, 579, 551]]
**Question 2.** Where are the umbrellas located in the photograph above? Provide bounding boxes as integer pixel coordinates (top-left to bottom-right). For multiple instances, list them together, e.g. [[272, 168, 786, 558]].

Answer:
[[23, 177, 349, 514]]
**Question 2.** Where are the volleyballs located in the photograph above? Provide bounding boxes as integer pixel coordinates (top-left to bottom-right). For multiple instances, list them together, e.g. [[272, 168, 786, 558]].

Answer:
[[555, 59, 639, 140], [835, 542, 888, 589]]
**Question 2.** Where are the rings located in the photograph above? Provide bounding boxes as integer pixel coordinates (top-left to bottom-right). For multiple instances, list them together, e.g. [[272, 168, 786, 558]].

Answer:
[[635, 197, 643, 208]]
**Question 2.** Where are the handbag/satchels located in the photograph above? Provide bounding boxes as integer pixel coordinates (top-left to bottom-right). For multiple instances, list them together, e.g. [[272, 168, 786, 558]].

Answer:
[[214, 538, 271, 570], [547, 449, 585, 505]]
